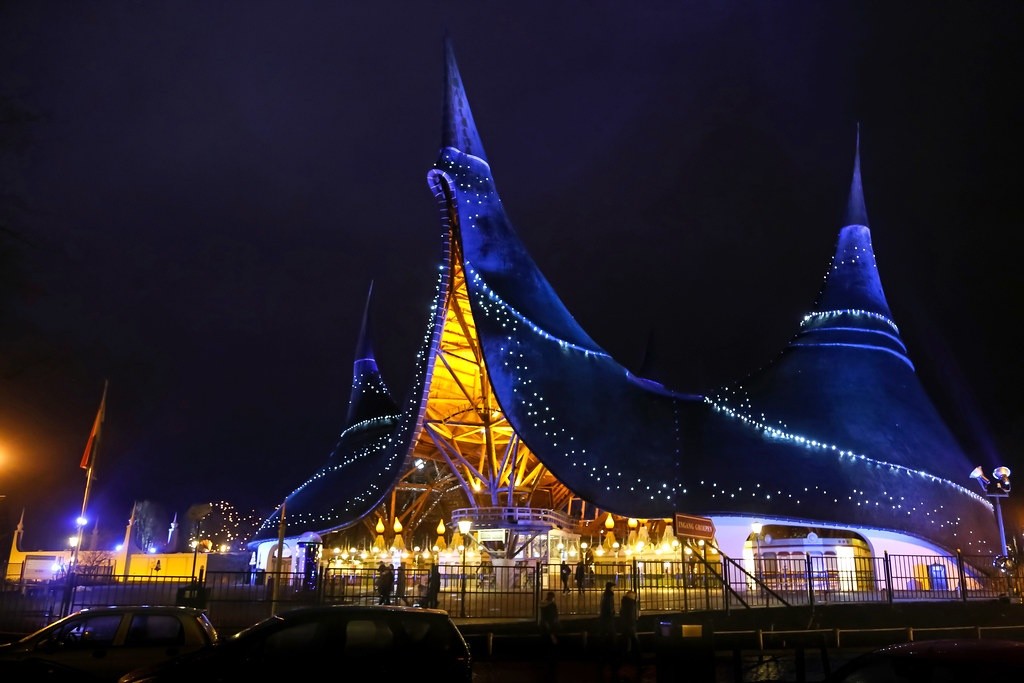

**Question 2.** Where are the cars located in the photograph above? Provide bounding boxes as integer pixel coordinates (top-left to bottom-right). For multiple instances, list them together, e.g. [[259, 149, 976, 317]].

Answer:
[[764, 637, 1024, 683], [115, 603, 473, 683], [0, 602, 219, 683]]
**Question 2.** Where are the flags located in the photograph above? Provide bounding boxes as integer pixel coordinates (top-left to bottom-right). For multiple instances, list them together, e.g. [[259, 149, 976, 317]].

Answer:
[[80, 399, 104, 481]]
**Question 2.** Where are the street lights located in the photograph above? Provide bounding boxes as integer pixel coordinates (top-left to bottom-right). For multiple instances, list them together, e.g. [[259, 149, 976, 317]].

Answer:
[[751, 521, 764, 598], [613, 542, 619, 586], [350, 547, 356, 568], [672, 541, 679, 586], [558, 543, 564, 566], [581, 542, 588, 566], [334, 548, 340, 567], [414, 547, 420, 570], [478, 546, 483, 581], [390, 547, 396, 566], [57, 536, 79, 619], [373, 548, 378, 567], [970, 466, 1013, 595], [433, 546, 439, 566], [456, 515, 472, 616]]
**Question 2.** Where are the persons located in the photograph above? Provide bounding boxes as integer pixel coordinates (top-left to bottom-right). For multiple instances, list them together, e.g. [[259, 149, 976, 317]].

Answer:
[[574, 562, 587, 593], [618, 585, 646, 668], [560, 562, 572, 594], [375, 561, 440, 610], [594, 581, 619, 649], [535, 590, 562, 655]]
